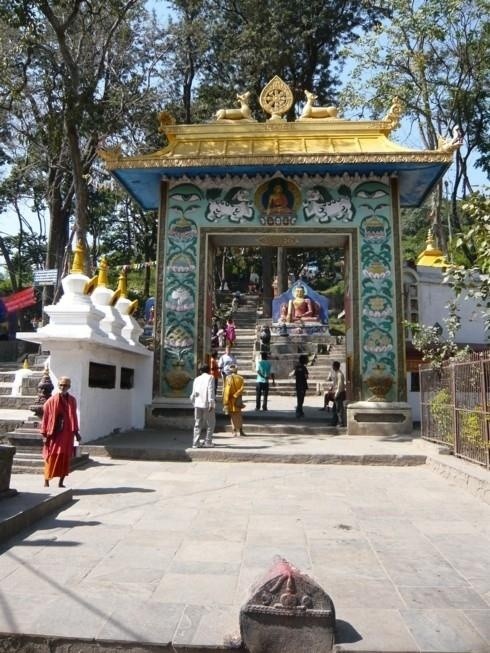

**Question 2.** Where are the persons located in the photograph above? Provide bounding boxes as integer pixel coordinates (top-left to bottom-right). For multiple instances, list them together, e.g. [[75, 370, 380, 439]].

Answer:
[[286, 285, 319, 324], [39, 376, 82, 488], [265, 184, 291, 215], [190, 297, 346, 449]]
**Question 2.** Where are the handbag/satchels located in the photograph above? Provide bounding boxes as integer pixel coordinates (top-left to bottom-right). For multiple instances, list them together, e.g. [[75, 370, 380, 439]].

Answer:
[[56, 417, 64, 432], [235, 395, 245, 408]]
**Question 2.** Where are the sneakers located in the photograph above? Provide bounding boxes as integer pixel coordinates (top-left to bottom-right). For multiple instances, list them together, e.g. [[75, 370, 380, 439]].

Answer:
[[327, 422, 343, 428], [192, 442, 214, 448]]
